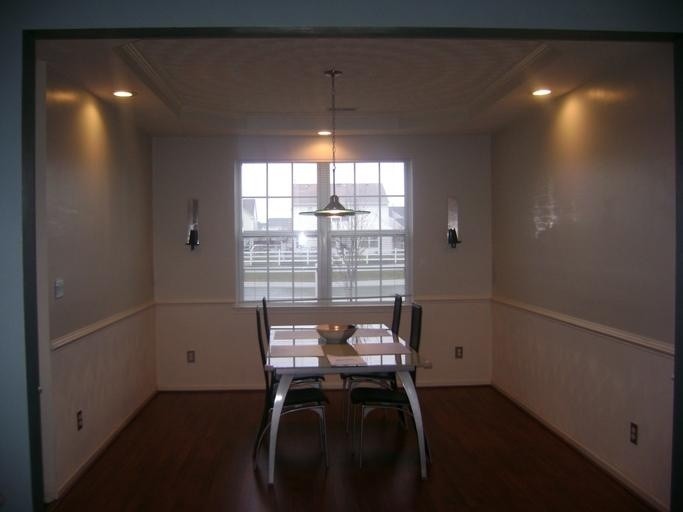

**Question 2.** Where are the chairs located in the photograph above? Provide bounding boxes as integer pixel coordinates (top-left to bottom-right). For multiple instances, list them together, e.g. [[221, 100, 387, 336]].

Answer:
[[354, 302, 434, 468], [341, 293, 408, 434], [251, 303, 330, 472], [262, 296, 327, 433]]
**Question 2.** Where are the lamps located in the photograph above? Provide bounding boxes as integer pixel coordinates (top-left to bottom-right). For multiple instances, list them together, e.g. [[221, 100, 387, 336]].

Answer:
[[299, 70, 372, 219]]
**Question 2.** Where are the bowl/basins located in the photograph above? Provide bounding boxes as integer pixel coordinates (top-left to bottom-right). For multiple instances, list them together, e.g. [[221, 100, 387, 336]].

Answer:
[[315, 323, 357, 342]]
